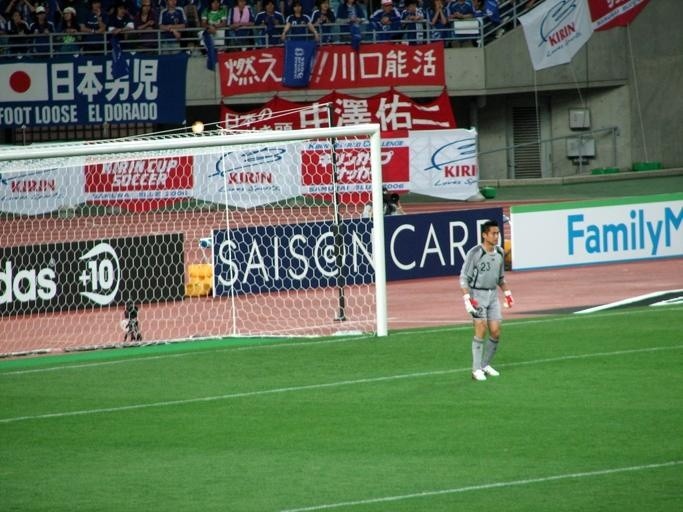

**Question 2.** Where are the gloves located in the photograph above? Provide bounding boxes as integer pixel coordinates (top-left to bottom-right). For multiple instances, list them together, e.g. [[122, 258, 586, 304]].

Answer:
[[463, 294, 479, 314], [503, 290, 513, 308]]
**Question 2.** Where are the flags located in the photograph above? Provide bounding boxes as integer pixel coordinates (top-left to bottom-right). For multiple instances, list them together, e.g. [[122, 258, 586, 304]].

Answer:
[[590, 1, 649, 33], [518, 1, 595, 72]]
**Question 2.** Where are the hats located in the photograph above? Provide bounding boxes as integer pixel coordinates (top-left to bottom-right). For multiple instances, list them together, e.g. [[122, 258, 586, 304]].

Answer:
[[35, 6, 45, 13], [383, 1, 392, 6]]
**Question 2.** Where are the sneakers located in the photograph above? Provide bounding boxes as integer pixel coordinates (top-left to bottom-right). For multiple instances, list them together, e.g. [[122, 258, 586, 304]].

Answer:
[[472, 365, 500, 382]]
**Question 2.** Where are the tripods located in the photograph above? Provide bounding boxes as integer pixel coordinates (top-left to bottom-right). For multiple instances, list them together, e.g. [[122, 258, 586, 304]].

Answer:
[[123, 318, 142, 341]]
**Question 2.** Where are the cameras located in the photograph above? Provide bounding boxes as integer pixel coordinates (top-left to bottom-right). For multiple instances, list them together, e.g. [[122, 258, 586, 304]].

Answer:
[[124, 305, 138, 318]]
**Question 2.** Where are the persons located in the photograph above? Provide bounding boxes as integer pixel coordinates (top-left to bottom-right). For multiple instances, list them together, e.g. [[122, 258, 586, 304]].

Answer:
[[1, 1, 201, 61], [456, 220, 515, 381], [121, 298, 142, 342], [361, 188, 406, 220], [200, 1, 501, 47]]
